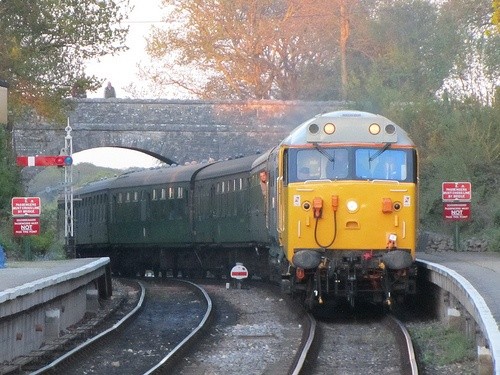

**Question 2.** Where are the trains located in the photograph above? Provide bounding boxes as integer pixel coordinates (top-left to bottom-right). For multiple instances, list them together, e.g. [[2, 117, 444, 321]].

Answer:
[[56, 109, 419, 312]]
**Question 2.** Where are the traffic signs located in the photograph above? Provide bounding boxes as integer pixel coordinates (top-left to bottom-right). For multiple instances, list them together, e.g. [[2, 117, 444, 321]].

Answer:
[[11, 196, 40, 237], [441, 181, 473, 221]]
